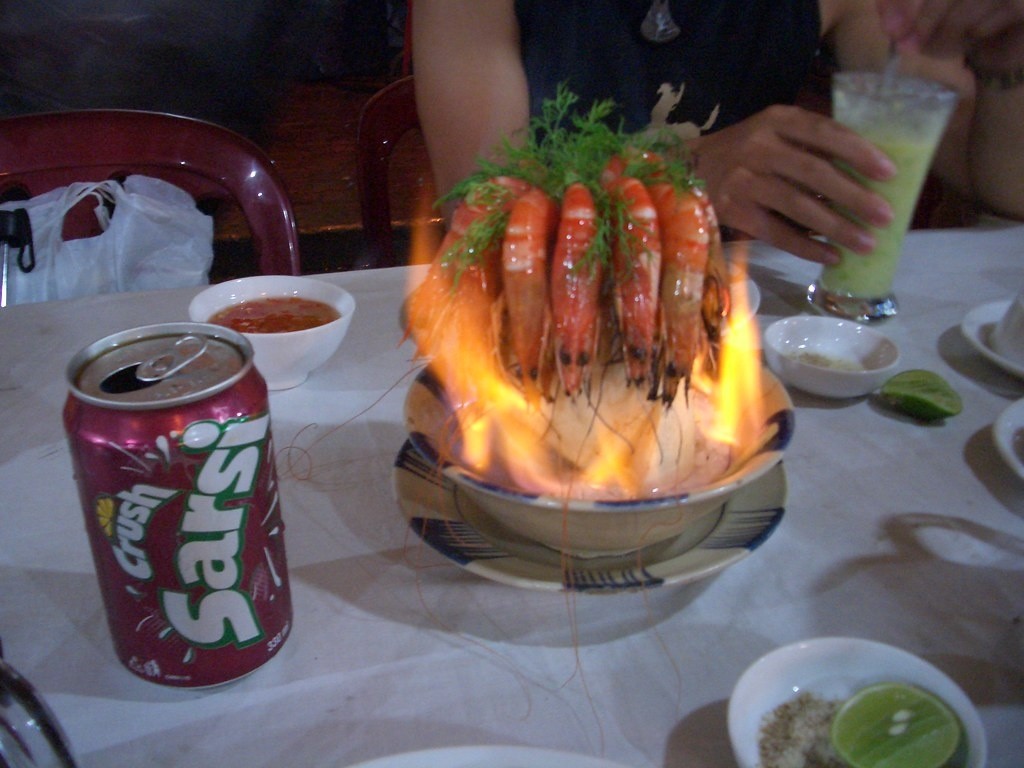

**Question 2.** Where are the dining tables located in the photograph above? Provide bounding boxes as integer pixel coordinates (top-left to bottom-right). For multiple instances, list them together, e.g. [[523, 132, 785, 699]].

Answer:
[[0, 236, 1024, 768]]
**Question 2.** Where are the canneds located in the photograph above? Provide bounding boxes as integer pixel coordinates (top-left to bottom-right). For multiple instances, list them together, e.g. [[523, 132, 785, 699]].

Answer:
[[62, 321, 292, 690]]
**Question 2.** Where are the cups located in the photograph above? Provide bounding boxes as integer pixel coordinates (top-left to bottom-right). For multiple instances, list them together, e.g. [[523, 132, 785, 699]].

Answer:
[[806, 73, 956, 320]]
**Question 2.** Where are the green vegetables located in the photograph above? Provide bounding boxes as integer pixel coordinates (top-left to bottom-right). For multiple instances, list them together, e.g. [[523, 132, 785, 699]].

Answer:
[[429, 76, 703, 294]]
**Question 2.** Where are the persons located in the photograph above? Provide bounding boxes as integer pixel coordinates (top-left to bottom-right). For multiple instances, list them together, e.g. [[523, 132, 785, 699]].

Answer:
[[411, 0, 1024, 266]]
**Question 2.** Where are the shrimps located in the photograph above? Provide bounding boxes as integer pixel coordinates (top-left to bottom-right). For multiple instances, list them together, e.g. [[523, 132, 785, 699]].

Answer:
[[394, 145, 729, 409]]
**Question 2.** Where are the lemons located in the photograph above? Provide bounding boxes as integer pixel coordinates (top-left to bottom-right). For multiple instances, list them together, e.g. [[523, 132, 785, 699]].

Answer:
[[830, 683, 962, 768], [879, 369, 964, 419]]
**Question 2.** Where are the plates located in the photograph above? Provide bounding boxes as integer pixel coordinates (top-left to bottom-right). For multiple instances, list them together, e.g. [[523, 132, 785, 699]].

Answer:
[[991, 397, 1024, 480], [960, 300, 1024, 378], [391, 434, 790, 596]]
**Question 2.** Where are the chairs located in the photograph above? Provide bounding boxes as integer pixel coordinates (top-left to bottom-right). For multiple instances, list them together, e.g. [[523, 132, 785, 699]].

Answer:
[[0, 109, 301, 301]]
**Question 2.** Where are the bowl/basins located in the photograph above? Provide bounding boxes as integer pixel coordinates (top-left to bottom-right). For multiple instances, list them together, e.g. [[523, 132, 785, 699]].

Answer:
[[764, 315, 900, 399], [405, 351, 797, 558], [188, 275, 355, 390], [725, 635, 987, 767]]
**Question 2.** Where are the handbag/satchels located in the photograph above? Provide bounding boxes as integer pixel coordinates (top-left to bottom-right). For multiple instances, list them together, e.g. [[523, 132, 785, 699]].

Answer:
[[0, 175, 214, 307]]
